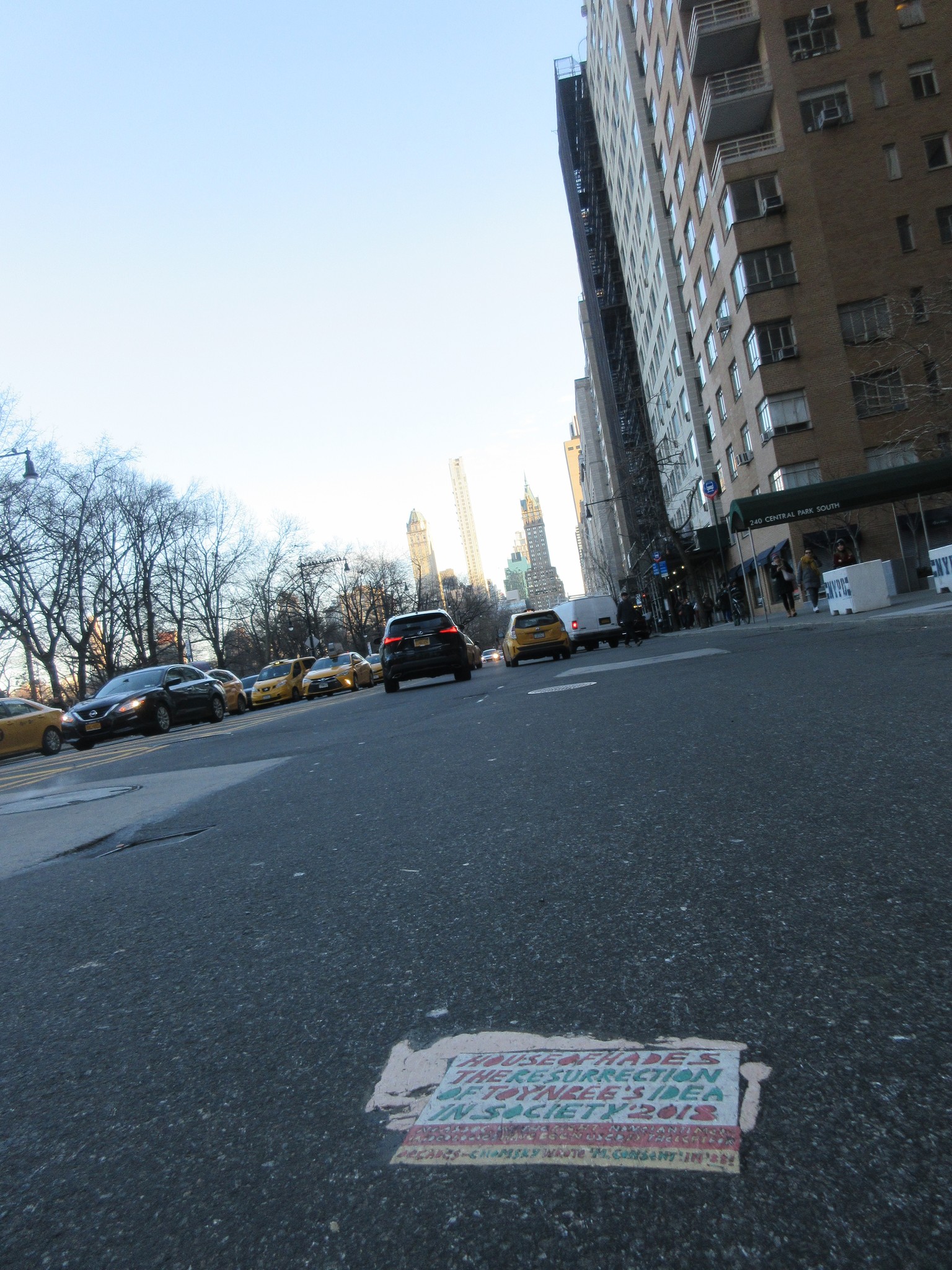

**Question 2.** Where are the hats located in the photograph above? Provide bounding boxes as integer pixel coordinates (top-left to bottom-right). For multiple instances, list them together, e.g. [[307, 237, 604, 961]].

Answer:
[[836, 539, 845, 547], [772, 553, 780, 560]]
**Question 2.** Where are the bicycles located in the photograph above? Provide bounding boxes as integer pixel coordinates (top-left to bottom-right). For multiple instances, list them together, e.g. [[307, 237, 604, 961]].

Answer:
[[721, 587, 751, 624]]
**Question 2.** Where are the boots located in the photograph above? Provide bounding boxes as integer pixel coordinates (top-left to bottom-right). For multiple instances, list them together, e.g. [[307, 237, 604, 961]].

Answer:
[[791, 609, 797, 616], [786, 610, 792, 617]]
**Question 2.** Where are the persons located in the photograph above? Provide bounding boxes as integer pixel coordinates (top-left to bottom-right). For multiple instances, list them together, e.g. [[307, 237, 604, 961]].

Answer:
[[797, 548, 822, 613], [833, 538, 856, 569], [674, 596, 694, 630], [701, 592, 714, 627], [646, 609, 656, 633], [715, 581, 743, 622], [770, 553, 797, 618], [616, 592, 643, 647]]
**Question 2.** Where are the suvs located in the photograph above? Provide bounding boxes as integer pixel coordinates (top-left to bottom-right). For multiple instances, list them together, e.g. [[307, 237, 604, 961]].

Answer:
[[548, 593, 622, 655], [373, 608, 471, 694]]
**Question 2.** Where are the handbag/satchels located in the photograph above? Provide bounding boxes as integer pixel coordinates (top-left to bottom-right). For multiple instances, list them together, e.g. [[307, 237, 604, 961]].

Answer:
[[781, 569, 795, 581]]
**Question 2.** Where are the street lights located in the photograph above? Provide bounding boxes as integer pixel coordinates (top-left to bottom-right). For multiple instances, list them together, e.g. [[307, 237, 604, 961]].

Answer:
[[584, 489, 675, 632], [363, 581, 409, 638], [458, 610, 483, 633], [288, 557, 350, 658], [420, 598, 451, 611]]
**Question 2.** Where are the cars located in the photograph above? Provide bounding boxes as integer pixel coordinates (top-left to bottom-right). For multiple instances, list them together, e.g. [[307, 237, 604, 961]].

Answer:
[[481, 649, 504, 663], [187, 660, 248, 725], [365, 652, 384, 686], [0, 697, 66, 760], [228, 672, 286, 716], [498, 608, 571, 667], [60, 664, 226, 750], [302, 642, 374, 701], [463, 634, 482, 670], [251, 656, 317, 709]]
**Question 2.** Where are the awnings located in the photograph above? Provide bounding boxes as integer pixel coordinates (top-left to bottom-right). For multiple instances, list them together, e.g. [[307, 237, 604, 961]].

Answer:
[[751, 545, 774, 570], [770, 538, 789, 562], [717, 564, 741, 585], [737, 556, 756, 576]]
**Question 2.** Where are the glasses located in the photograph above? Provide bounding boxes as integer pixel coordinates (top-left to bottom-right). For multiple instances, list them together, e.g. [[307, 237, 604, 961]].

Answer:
[[705, 595, 708, 596], [805, 552, 811, 554]]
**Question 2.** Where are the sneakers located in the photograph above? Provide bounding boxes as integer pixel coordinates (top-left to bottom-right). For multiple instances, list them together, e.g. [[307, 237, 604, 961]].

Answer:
[[637, 640, 642, 646], [625, 644, 631, 648]]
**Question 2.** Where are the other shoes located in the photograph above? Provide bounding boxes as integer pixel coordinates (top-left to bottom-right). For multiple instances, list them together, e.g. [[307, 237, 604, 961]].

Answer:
[[813, 607, 819, 612]]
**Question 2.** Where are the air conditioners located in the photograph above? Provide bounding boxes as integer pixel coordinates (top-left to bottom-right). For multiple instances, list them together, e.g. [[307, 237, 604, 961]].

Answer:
[[762, 195, 783, 216], [810, 5, 832, 25], [715, 316, 732, 332], [779, 346, 796, 361], [817, 107, 842, 129], [760, 432, 771, 444], [735, 451, 751, 466]]
